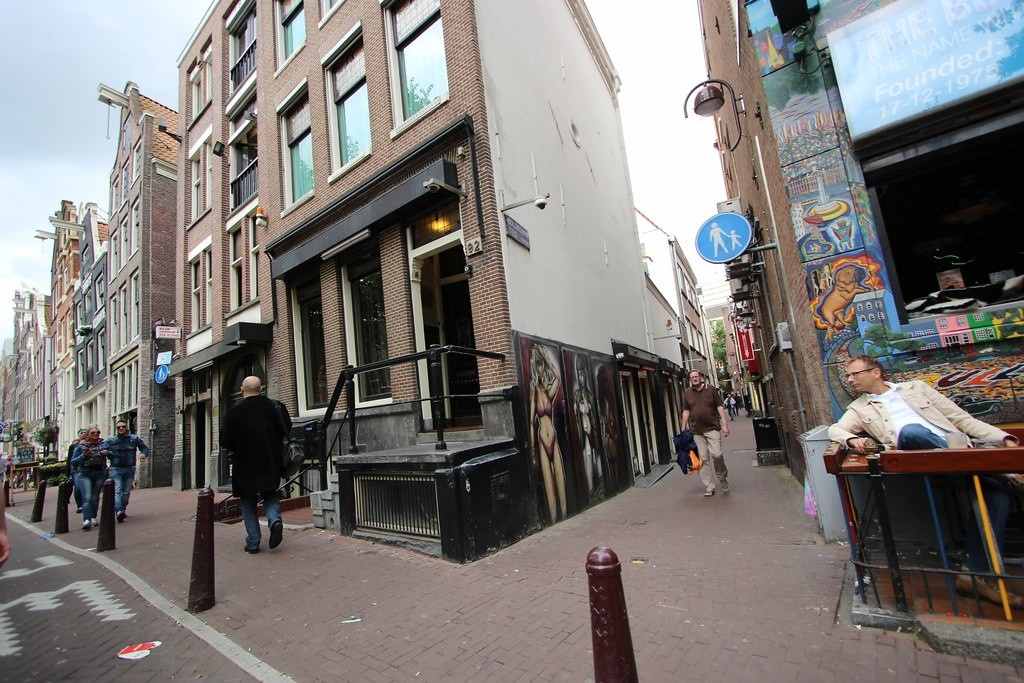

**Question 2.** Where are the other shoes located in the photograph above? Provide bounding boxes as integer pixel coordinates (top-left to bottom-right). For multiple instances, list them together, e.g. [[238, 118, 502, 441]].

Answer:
[[245, 545, 259, 554], [91, 518, 99, 527], [82, 520, 91, 530], [269, 521, 283, 549], [988, 473, 1024, 489], [956, 575, 1024, 610], [704, 490, 714, 496], [77, 508, 82, 513], [117, 511, 127, 523], [720, 478, 729, 492]]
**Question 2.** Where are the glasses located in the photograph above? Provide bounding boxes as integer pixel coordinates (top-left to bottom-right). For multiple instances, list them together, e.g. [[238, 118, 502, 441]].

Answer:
[[845, 368, 877, 379], [116, 425, 126, 429], [88, 430, 100, 434], [80, 432, 87, 434]]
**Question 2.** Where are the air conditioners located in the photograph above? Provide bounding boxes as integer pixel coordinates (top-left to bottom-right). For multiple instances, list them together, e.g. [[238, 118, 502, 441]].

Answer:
[[717, 198, 752, 301]]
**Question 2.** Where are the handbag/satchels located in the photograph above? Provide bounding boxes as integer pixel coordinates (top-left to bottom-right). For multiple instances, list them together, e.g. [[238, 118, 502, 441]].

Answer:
[[273, 399, 305, 479], [687, 450, 702, 471]]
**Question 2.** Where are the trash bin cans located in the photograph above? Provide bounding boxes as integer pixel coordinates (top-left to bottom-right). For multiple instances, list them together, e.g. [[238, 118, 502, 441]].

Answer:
[[753, 417, 780, 452]]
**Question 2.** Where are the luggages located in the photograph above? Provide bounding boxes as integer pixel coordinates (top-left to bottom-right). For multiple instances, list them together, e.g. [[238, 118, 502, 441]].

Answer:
[[58, 477, 74, 504]]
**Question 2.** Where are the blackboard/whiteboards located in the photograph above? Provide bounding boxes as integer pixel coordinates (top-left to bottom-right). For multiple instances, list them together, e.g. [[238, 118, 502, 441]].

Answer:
[[16, 444, 35, 463]]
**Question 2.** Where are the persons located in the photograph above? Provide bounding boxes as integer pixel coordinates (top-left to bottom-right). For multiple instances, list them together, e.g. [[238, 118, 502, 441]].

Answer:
[[0, 452, 13, 568], [70, 427, 113, 530], [220, 376, 294, 553], [827, 356, 1024, 607], [102, 420, 149, 522], [724, 394, 736, 420], [680, 369, 730, 495], [65, 429, 88, 513], [734, 393, 740, 416]]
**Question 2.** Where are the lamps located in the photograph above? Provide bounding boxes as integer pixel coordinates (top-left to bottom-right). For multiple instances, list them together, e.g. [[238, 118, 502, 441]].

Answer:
[[740, 306, 761, 331], [256, 207, 269, 228], [685, 78, 749, 152], [213, 141, 229, 160]]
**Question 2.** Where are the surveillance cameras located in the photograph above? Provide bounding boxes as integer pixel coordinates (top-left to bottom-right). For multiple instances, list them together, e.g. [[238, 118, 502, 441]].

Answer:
[[535, 198, 548, 209], [428, 184, 439, 192]]
